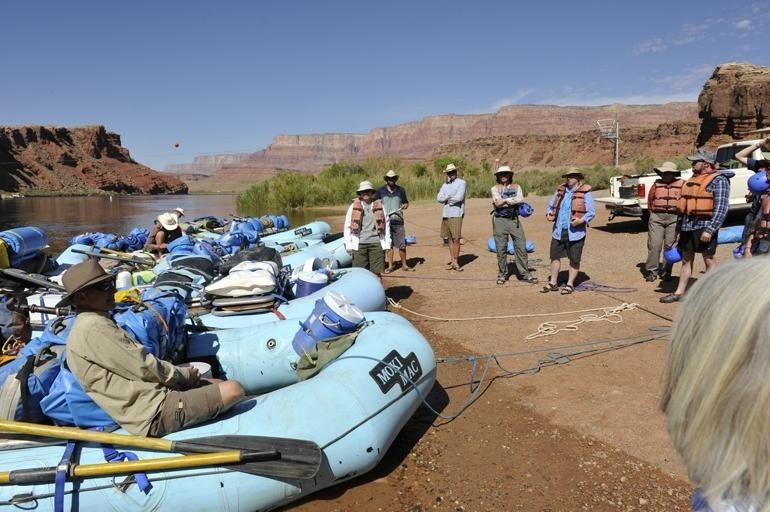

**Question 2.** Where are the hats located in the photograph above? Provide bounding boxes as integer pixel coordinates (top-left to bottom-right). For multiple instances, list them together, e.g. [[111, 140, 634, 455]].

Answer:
[[383, 170, 399, 181], [443, 163, 460, 173], [687, 146, 717, 164], [356, 181, 376, 194], [55, 259, 118, 309], [653, 162, 681, 177], [173, 207, 185, 216], [494, 166, 514, 175], [157, 212, 178, 231], [562, 167, 585, 179]]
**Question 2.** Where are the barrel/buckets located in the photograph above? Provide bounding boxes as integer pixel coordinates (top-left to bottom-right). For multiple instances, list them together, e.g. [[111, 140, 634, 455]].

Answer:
[[132, 269, 154, 286], [291, 289, 364, 358], [294, 271, 328, 298], [115, 271, 133, 289]]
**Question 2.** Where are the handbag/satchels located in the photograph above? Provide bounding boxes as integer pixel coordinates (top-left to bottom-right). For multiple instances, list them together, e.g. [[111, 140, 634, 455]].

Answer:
[[41, 316, 75, 343], [733, 237, 770, 258], [167, 234, 198, 252], [0, 344, 66, 425]]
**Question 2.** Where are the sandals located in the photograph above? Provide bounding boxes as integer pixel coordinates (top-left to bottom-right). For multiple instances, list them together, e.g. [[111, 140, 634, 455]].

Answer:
[[561, 284, 575, 295], [497, 276, 505, 284], [540, 283, 558, 292], [527, 277, 538, 284]]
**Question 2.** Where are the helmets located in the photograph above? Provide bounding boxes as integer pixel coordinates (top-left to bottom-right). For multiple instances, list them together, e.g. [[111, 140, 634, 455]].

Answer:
[[748, 171, 769, 193], [664, 247, 681, 262]]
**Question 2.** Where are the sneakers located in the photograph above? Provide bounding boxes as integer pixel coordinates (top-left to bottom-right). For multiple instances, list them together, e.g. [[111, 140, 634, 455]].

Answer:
[[660, 293, 681, 303]]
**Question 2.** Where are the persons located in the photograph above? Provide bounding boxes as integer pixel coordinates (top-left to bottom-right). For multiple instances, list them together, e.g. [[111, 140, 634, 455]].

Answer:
[[540, 166, 595, 295], [172, 207, 185, 219], [643, 161, 686, 283], [436, 163, 467, 272], [344, 180, 392, 278], [734, 137, 770, 258], [54, 259, 247, 439], [659, 146, 730, 303], [374, 170, 415, 274], [491, 166, 538, 285], [144, 212, 182, 258]]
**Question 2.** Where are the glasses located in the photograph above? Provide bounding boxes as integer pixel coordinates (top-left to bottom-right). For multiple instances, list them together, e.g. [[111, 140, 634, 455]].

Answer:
[[94, 278, 116, 292]]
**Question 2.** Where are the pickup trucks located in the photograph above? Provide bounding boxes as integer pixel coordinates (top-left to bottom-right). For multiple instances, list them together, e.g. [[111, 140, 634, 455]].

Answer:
[[592, 138, 770, 227]]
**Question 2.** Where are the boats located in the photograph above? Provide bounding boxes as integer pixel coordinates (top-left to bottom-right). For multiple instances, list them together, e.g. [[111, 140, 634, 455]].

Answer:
[[0, 220, 387, 365], [0, 309, 437, 512]]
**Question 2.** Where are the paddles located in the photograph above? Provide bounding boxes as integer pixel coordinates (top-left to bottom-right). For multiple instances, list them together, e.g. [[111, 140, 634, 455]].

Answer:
[[1, 420, 320, 480]]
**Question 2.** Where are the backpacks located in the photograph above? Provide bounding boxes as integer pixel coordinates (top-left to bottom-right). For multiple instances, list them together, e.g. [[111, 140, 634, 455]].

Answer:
[[494, 188, 517, 217]]
